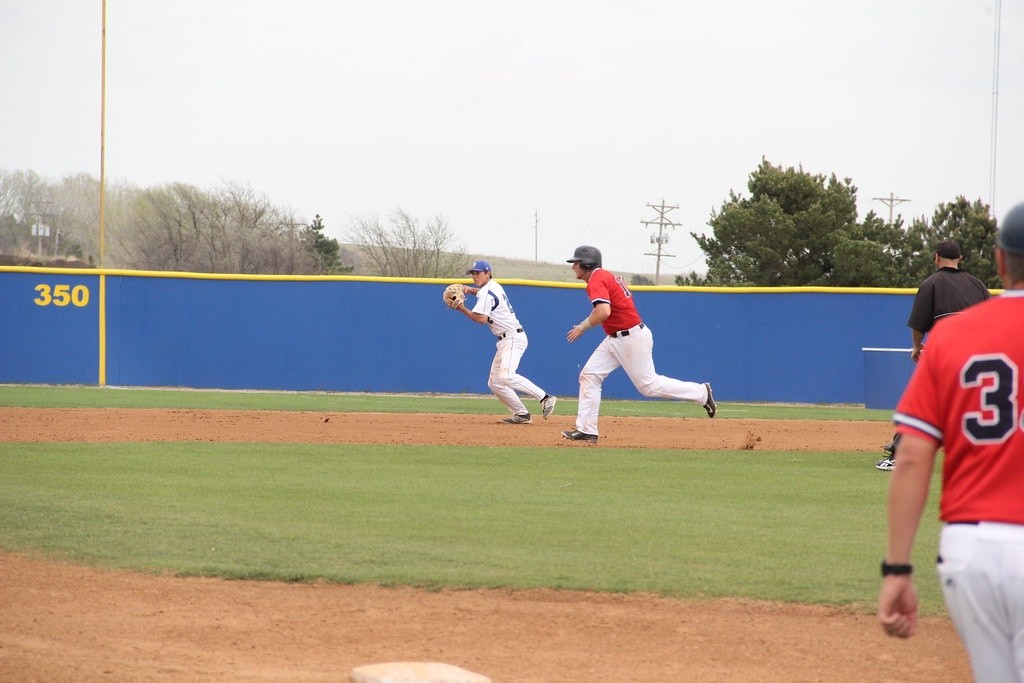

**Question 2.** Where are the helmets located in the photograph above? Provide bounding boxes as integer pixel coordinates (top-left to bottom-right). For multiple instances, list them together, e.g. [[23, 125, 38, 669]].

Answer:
[[566, 245, 602, 269]]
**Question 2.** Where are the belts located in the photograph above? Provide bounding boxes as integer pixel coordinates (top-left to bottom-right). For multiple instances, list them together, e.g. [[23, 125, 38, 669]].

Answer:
[[609, 323, 645, 338], [497, 328, 525, 340]]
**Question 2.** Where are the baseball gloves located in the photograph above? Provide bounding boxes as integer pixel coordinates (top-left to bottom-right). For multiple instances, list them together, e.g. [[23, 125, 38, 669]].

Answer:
[[443, 283, 465, 310]]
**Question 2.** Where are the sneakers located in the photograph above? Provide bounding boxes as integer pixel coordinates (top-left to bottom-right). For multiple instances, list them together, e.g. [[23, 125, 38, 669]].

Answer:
[[499, 413, 533, 424], [560, 429, 598, 443], [874, 457, 898, 471], [702, 382, 717, 419], [541, 394, 557, 419]]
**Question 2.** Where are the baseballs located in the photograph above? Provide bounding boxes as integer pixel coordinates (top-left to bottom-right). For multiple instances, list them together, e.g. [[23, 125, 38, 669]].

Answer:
[[464, 284, 468, 293]]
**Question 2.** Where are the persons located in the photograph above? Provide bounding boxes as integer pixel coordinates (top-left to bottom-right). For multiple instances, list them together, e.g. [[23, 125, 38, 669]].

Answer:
[[447, 259, 558, 424], [878, 199, 1024, 683], [559, 246, 718, 440], [874, 240, 993, 471]]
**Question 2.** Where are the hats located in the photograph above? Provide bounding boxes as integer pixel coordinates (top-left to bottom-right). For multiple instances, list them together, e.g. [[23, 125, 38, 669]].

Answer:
[[465, 260, 491, 276], [996, 201, 1024, 256], [933, 240, 961, 261]]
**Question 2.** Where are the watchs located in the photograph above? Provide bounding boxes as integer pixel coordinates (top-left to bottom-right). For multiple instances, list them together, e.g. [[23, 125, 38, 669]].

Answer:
[[880, 560, 912, 576]]
